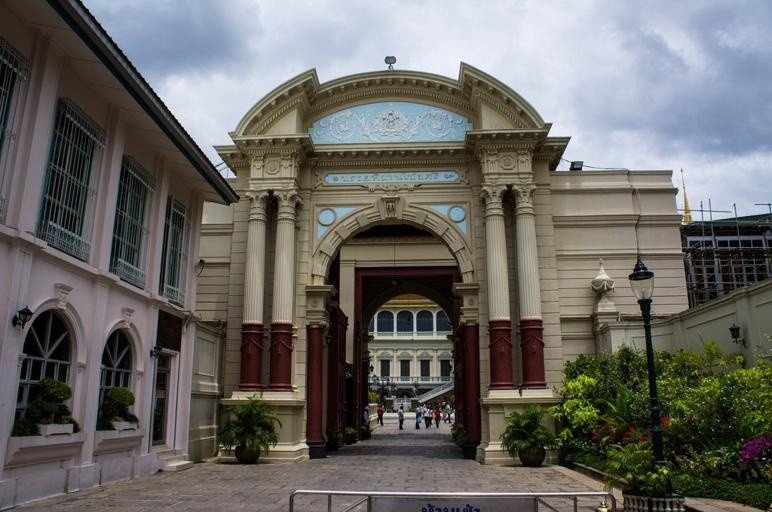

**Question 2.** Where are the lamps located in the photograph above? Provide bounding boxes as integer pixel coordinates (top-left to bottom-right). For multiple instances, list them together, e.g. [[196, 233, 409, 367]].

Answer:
[[570, 160, 584, 170], [729, 323, 746, 346], [149, 342, 161, 357], [12, 305, 34, 330]]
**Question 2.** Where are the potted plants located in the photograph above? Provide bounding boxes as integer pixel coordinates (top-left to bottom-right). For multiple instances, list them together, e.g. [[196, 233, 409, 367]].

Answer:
[[105, 387, 139, 430], [499, 404, 555, 467], [216, 395, 282, 463], [326, 428, 339, 451], [345, 428, 357, 445], [25, 378, 75, 435]]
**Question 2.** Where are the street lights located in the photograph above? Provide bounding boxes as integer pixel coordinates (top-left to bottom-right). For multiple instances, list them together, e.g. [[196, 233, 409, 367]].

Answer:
[[628, 254, 668, 467]]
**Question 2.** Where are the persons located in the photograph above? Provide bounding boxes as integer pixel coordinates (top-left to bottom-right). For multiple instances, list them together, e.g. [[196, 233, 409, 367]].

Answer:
[[364, 406, 372, 440], [415, 400, 452, 430], [399, 403, 403, 412], [398, 406, 404, 429], [376, 404, 384, 427]]
